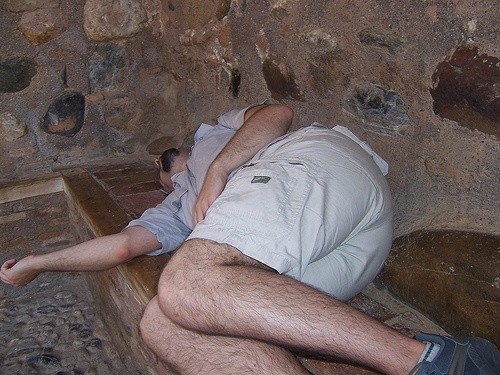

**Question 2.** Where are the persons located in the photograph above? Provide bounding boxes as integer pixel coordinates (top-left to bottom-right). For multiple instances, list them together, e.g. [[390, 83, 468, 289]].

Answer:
[[0, 101, 499, 375]]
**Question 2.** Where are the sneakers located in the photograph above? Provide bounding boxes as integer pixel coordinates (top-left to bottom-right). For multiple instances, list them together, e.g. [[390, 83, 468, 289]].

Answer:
[[408, 332, 500, 375]]
